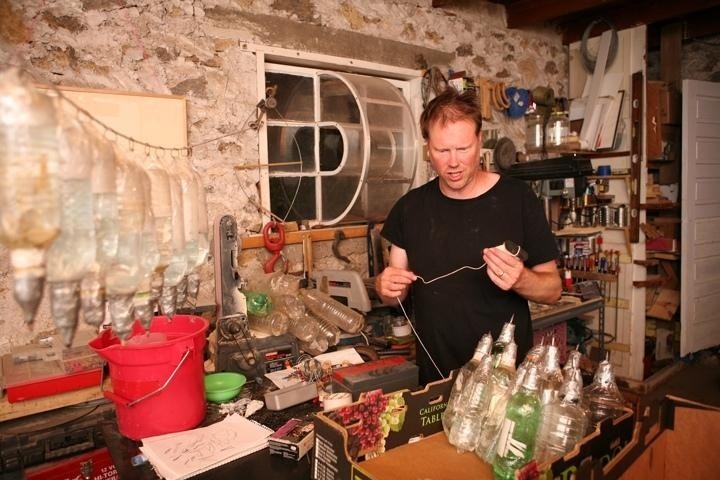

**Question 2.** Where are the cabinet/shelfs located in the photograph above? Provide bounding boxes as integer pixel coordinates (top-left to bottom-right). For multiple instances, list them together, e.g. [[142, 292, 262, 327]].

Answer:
[[644, 160, 682, 263], [587, 174, 632, 259]]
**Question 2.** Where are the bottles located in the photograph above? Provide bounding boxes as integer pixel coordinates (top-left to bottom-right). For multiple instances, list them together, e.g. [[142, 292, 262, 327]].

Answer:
[[544, 110, 571, 150], [241, 272, 365, 357], [526, 113, 546, 153], [0, 71, 212, 347], [440, 322, 624, 480]]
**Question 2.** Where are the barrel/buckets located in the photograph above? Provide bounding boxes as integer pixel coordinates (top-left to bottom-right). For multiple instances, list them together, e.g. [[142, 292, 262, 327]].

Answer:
[[546, 105, 570, 150], [88, 318, 210, 441], [526, 114, 543, 151]]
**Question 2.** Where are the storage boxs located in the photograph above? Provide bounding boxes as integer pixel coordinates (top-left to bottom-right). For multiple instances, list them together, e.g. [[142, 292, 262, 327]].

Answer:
[[312, 371, 634, 480], [572, 394, 720, 480]]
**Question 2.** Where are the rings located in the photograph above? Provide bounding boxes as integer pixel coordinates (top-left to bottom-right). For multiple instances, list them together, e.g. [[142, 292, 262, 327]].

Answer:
[[498, 272, 504, 277]]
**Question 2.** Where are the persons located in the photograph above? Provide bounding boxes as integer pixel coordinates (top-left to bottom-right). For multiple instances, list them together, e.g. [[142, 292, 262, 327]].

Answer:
[[371, 88, 562, 390]]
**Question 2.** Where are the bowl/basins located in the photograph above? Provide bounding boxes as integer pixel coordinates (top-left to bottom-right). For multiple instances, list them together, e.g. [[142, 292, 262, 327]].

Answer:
[[203, 371, 246, 403]]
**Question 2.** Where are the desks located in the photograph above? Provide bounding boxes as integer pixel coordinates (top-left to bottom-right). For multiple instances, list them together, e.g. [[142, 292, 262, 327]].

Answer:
[[404, 297, 606, 389], [98, 394, 357, 480]]
